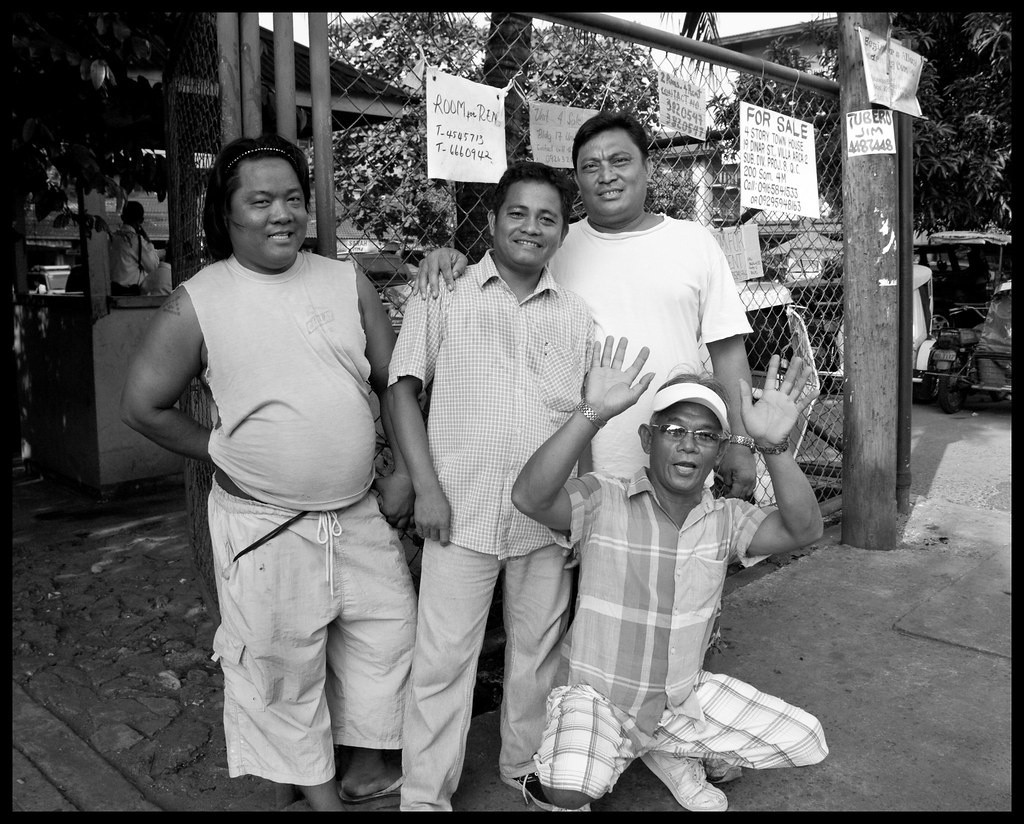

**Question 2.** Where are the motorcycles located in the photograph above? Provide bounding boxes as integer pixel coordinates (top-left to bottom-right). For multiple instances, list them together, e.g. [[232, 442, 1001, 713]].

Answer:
[[928, 302, 991, 414]]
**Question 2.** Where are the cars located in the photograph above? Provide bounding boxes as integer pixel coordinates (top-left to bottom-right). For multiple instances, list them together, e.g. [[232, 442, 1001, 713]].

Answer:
[[25, 266, 73, 293]]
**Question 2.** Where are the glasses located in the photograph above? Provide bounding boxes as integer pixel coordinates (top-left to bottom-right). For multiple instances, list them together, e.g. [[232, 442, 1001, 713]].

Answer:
[[650, 424, 727, 447]]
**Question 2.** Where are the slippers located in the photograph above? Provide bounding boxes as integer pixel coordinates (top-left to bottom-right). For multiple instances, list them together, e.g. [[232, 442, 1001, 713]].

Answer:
[[340, 775, 402, 802]]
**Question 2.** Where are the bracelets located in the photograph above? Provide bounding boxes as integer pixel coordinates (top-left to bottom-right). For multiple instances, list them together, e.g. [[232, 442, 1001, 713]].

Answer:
[[754, 435, 792, 455], [730, 431, 757, 454], [575, 398, 607, 429]]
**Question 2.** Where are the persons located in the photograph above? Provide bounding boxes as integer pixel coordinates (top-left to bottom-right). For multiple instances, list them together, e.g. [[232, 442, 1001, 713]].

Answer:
[[116, 128, 418, 811], [387, 161, 595, 811], [413, 114, 757, 785], [511, 335, 829, 811], [109, 200, 172, 296]]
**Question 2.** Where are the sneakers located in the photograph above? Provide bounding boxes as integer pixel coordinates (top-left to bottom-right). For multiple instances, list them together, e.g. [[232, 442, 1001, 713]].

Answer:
[[643, 750, 729, 813], [500, 773, 589, 812], [703, 756, 743, 783]]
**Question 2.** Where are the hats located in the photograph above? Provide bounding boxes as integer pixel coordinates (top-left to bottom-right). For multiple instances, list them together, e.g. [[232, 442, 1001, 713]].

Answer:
[[652, 383, 728, 430]]
